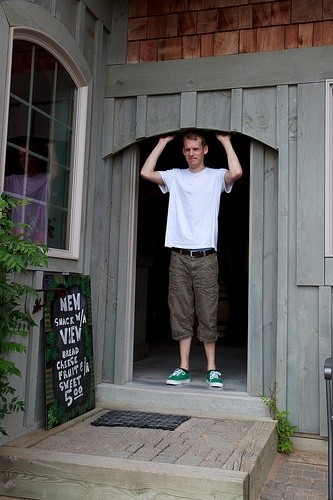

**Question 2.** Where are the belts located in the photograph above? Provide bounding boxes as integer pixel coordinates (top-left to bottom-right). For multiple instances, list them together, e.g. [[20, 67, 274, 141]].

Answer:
[[172, 247, 215, 257]]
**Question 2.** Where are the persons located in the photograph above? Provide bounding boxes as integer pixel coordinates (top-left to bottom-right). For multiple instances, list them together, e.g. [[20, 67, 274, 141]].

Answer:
[[4, 142, 61, 248], [140, 129, 242, 388]]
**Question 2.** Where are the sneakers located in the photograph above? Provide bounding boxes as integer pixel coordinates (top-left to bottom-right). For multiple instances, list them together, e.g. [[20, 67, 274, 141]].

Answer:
[[165, 368, 190, 384], [206, 371, 223, 387]]
